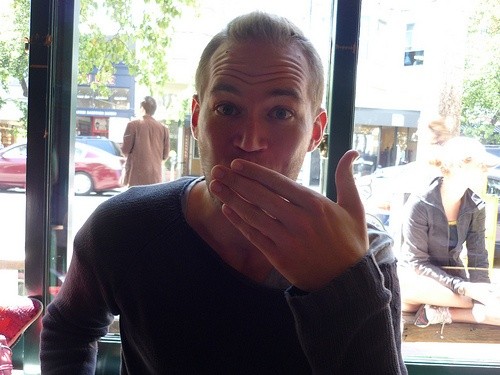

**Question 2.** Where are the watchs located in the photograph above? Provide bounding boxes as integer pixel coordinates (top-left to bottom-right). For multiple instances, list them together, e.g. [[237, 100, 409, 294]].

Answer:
[[457, 282, 467, 297]]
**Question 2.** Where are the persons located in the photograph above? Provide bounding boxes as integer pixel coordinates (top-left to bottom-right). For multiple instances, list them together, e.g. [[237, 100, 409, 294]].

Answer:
[[39, 11, 410, 375], [392, 136, 500, 329], [121, 95, 169, 189], [94, 121, 105, 132]]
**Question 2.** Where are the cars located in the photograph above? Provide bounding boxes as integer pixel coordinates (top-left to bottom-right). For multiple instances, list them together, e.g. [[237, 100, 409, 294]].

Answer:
[[355, 146, 500, 259], [75, 136, 124, 156], [0, 139, 126, 196]]
